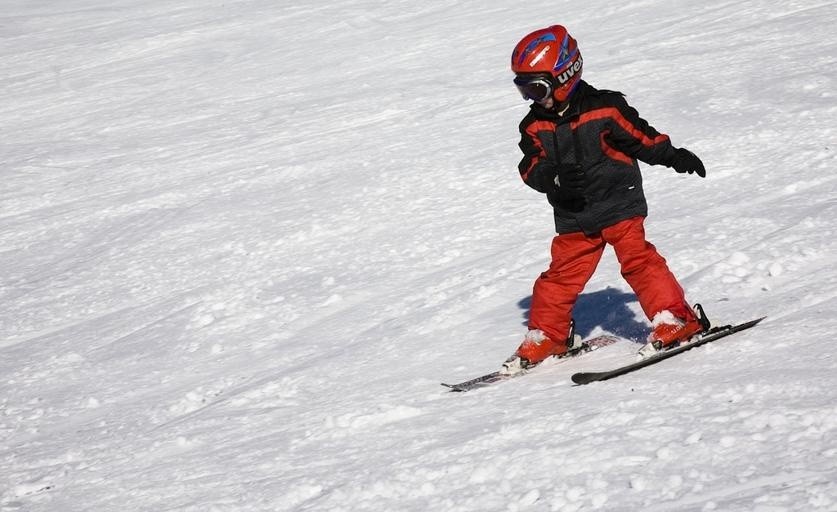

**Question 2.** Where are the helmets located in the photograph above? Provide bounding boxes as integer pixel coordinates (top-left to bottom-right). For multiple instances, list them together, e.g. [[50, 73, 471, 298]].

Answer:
[[511, 24, 588, 105]]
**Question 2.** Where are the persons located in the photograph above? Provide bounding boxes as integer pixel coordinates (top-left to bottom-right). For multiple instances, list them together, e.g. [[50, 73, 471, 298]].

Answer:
[[499, 25, 705, 376]]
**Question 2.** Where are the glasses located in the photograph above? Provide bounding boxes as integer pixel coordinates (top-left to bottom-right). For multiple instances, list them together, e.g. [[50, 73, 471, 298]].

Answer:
[[513, 78, 553, 102]]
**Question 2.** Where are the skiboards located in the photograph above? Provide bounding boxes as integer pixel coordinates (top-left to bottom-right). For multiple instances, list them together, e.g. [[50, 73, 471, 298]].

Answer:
[[441, 315, 767, 391]]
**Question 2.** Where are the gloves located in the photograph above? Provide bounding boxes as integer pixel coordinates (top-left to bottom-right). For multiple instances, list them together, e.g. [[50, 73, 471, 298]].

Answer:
[[662, 142, 708, 182], [555, 162, 588, 195]]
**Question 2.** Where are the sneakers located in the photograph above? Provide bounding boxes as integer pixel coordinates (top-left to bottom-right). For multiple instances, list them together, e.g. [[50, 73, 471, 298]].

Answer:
[[511, 325, 572, 367], [647, 303, 706, 351]]
